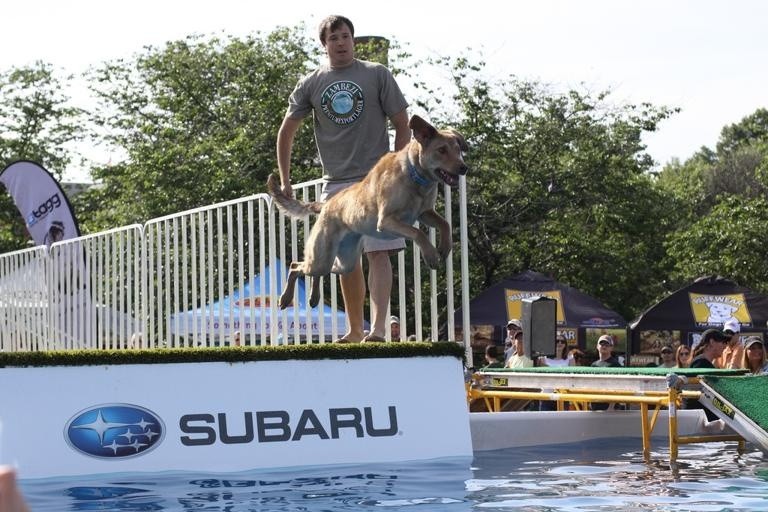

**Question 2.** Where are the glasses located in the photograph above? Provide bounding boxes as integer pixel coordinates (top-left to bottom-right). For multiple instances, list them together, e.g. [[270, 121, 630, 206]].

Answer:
[[556, 340, 565, 344], [679, 352, 689, 356]]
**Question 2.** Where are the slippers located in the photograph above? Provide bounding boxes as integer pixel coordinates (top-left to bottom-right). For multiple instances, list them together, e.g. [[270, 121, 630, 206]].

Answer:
[[360, 335, 386, 343], [333, 335, 350, 343]]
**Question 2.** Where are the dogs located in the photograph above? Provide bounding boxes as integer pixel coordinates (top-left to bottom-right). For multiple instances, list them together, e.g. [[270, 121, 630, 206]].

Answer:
[[266, 114, 471, 311]]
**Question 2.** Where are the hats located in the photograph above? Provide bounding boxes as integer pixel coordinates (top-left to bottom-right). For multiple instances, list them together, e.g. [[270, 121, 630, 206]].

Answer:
[[743, 335, 763, 348], [702, 328, 732, 344], [505, 318, 523, 340], [390, 315, 400, 326], [722, 316, 741, 334], [598, 335, 613, 346], [659, 344, 673, 353]]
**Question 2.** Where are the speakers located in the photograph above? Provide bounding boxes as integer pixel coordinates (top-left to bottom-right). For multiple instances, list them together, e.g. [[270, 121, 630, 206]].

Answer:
[[521, 294, 558, 359]]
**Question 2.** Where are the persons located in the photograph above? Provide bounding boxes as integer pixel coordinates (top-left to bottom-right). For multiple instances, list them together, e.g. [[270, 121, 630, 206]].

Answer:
[[466, 319, 768, 413], [275, 15, 412, 343]]
